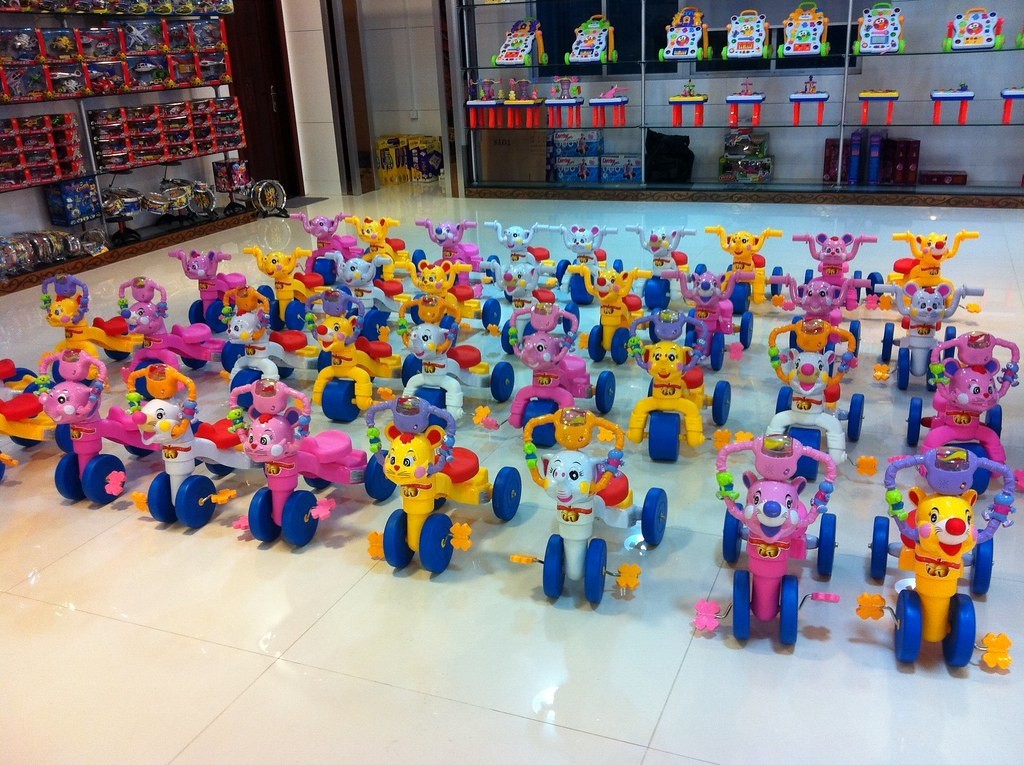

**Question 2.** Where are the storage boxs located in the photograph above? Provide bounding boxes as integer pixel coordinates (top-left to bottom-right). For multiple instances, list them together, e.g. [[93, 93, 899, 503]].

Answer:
[[0, 0, 248, 228], [529, 130, 642, 184], [376, 133, 443, 186]]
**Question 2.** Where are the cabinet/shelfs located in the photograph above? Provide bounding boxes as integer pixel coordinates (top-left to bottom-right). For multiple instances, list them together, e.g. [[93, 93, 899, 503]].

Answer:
[[458, 0, 1024, 197], [0, 11, 257, 297]]
